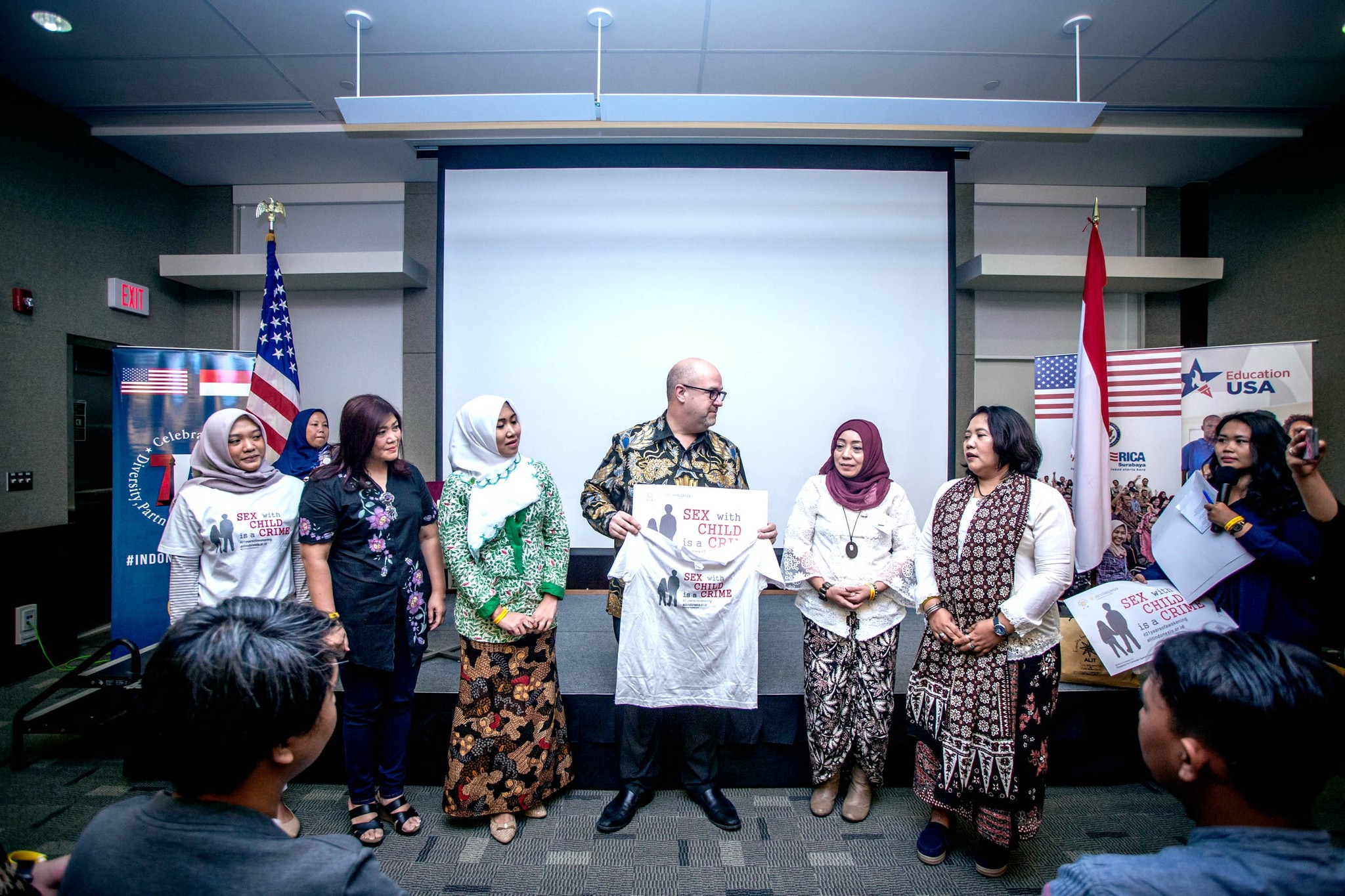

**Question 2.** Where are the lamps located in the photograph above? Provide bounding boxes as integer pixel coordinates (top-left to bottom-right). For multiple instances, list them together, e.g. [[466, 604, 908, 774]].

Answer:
[[106, 277, 151, 317]]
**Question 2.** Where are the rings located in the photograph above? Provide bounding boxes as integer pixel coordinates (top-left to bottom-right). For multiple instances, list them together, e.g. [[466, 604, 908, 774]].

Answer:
[[938, 633, 945, 638], [524, 621, 531, 628], [1289, 448, 1296, 456], [967, 635, 975, 649]]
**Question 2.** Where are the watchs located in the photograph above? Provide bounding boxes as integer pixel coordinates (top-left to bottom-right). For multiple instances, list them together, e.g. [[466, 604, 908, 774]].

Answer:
[[993, 616, 1013, 640], [1230, 519, 1249, 535]]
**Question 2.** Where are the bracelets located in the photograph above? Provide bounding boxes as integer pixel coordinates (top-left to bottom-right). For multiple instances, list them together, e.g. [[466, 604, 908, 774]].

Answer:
[[328, 612, 340, 620], [923, 602, 947, 621], [1223, 516, 1245, 532], [865, 583, 879, 601], [817, 582, 832, 602], [491, 606, 510, 624]]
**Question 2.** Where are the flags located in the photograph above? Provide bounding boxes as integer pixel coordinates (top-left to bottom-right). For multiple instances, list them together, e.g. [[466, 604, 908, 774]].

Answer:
[[244, 230, 301, 467], [1069, 216, 1113, 574]]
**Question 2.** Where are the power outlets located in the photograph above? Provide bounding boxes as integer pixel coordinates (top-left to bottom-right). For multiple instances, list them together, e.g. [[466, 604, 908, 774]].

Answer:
[[15, 603, 40, 645]]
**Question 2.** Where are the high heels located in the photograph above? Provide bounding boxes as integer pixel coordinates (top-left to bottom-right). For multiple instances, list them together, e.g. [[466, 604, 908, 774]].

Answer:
[[347, 796, 384, 848], [373, 795, 422, 836]]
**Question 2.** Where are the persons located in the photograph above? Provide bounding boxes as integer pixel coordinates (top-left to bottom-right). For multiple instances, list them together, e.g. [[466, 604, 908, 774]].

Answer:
[[1037, 471, 1175, 618], [58, 599, 409, 896], [435, 395, 572, 841], [1182, 414, 1222, 488], [1282, 424, 1345, 567], [579, 356, 779, 835], [1281, 414, 1313, 440], [1132, 411, 1325, 648], [787, 417, 921, 821], [1039, 627, 1345, 896], [298, 394, 447, 846], [909, 404, 1076, 879], [158, 405, 341, 837], [0, 838, 72, 896]]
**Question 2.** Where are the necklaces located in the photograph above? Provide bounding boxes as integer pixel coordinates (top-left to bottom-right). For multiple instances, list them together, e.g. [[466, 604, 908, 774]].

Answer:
[[975, 479, 993, 506], [842, 504, 861, 558]]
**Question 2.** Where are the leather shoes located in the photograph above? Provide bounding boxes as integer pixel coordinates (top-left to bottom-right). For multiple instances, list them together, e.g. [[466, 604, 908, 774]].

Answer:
[[596, 788, 654, 832], [688, 786, 741, 830]]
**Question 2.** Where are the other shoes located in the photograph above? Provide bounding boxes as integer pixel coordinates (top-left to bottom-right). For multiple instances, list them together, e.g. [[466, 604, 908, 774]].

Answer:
[[974, 839, 1009, 875], [916, 813, 957, 865], [272, 799, 301, 838], [524, 800, 549, 817], [489, 815, 517, 845]]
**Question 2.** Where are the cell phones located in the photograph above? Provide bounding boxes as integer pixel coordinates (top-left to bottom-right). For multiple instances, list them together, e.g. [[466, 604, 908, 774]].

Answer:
[[1296, 428, 1319, 461]]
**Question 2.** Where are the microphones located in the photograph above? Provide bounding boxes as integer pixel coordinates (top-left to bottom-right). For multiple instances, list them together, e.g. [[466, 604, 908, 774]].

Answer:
[[1210, 466, 1240, 533]]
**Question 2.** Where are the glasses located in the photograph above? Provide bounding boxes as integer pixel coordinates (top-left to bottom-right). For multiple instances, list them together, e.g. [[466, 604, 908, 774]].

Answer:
[[683, 383, 727, 402]]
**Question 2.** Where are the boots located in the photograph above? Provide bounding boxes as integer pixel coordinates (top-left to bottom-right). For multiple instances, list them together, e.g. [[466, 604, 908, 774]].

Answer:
[[809, 767, 841, 816], [842, 764, 872, 820]]
